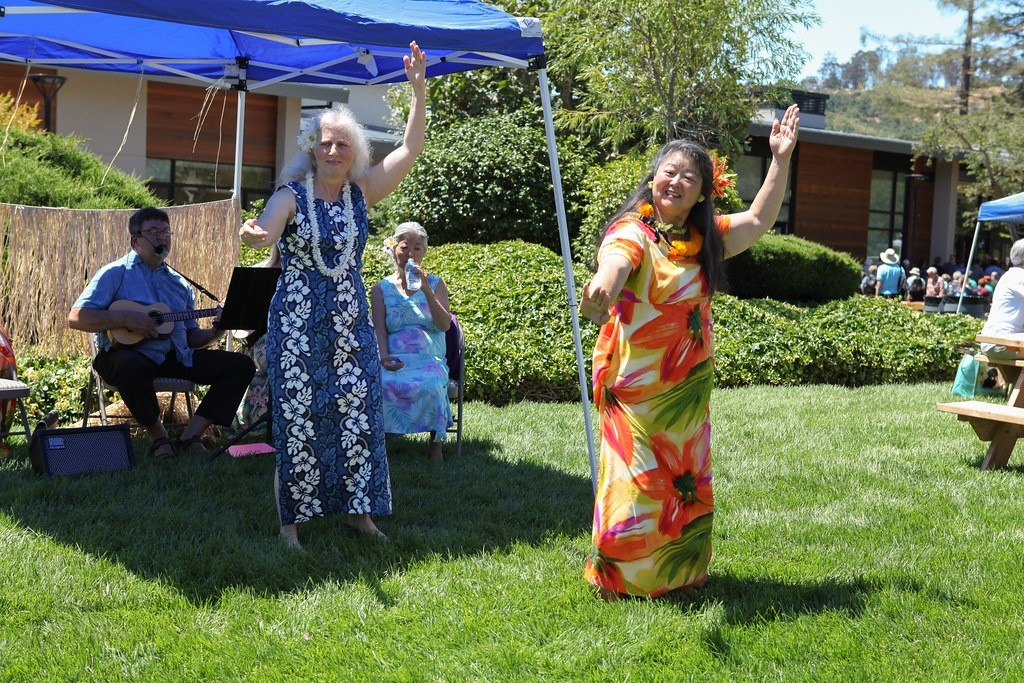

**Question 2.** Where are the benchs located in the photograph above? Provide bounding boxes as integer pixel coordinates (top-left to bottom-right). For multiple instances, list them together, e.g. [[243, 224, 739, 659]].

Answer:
[[974, 355, 1024, 368], [937, 401, 1024, 425]]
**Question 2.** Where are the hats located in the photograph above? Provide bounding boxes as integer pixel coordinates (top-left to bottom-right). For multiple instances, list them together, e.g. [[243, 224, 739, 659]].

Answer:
[[880, 249, 900, 264], [910, 267, 920, 276]]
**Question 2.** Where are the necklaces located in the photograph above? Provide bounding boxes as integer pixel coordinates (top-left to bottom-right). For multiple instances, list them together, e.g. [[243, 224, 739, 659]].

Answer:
[[655, 221, 687, 234], [305, 169, 356, 277]]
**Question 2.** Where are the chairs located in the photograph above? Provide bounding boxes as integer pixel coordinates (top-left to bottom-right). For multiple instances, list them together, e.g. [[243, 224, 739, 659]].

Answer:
[[0, 332, 32, 446], [82, 331, 194, 450], [443, 313, 465, 457]]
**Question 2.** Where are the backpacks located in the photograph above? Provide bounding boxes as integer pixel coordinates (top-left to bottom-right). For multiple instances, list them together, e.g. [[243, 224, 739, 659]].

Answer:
[[909, 278, 922, 296]]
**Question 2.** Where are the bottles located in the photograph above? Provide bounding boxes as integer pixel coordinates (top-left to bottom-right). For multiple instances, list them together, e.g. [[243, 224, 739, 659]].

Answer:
[[405, 258, 421, 290]]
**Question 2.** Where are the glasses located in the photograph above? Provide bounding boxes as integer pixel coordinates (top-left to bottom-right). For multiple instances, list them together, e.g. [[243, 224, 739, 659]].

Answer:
[[133, 227, 174, 238]]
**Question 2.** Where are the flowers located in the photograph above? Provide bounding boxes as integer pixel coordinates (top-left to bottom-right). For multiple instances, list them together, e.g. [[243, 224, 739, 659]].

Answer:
[[711, 152, 737, 198]]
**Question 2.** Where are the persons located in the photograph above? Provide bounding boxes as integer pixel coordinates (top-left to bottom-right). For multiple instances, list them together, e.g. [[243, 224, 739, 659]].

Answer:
[[577, 104, 799, 601], [981, 239, 1024, 358], [370, 222, 452, 462], [861, 264, 877, 294], [240, 40, 426, 552], [874, 248, 905, 298], [69, 207, 256, 456], [903, 255, 1004, 302]]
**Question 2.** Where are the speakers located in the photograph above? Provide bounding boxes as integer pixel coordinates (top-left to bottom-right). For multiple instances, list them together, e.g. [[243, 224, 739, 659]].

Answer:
[[28, 423, 136, 478]]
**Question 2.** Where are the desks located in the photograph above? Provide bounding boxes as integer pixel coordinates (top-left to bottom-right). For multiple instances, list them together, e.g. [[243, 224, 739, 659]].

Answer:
[[975, 333, 1024, 470]]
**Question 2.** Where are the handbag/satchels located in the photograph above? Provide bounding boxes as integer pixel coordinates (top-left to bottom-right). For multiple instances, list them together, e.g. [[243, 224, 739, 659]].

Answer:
[[951, 353, 1009, 400]]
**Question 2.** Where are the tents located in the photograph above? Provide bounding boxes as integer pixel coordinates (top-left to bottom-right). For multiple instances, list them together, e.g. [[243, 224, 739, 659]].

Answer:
[[0, 0, 597, 496], [956, 192, 1024, 312]]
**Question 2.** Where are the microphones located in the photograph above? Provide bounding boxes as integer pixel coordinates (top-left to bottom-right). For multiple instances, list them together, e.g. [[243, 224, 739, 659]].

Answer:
[[136, 232, 163, 254]]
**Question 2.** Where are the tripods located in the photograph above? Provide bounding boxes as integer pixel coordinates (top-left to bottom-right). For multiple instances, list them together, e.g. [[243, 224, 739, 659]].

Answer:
[[206, 267, 287, 460]]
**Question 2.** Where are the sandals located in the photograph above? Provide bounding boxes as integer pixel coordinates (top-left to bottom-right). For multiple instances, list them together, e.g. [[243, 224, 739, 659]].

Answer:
[[149, 437, 174, 458], [176, 435, 207, 456]]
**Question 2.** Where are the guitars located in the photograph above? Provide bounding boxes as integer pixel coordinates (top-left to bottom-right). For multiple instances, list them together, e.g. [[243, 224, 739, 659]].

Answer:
[[106, 299, 221, 350]]
